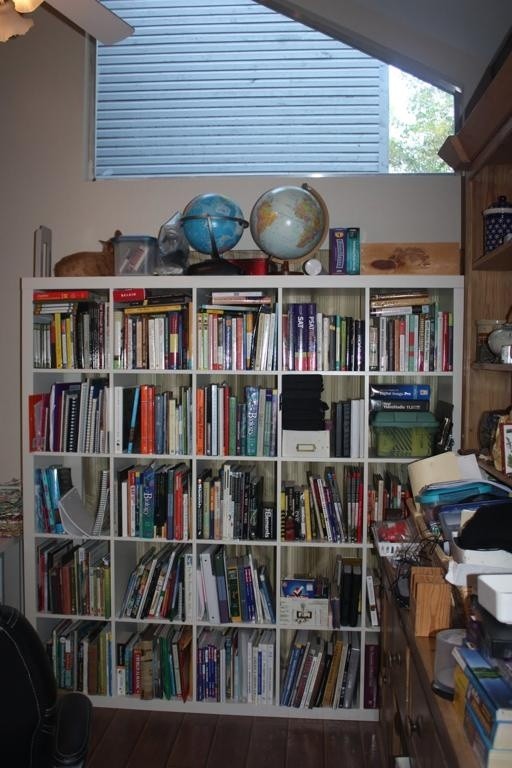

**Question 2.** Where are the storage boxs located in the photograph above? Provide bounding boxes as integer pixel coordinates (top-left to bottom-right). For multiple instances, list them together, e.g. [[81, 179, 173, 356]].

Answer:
[[112, 235, 158, 275]]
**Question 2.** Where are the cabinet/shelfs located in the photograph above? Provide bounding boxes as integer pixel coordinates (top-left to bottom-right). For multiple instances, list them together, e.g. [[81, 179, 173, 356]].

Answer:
[[20, 275, 464, 721], [371, 29, 511, 768]]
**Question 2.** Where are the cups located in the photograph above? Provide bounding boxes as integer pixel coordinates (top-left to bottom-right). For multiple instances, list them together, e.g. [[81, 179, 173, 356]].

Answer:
[[500, 344, 512, 364], [431, 627, 466, 693]]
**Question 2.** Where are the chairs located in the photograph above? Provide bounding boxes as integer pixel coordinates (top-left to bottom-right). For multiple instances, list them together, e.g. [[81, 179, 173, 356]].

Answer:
[[0, 604, 92, 767]]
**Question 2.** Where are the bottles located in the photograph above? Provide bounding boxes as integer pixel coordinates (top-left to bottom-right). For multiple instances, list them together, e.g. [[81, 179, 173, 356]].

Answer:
[[474, 318, 507, 364], [483, 195, 511, 255], [487, 322, 511, 363]]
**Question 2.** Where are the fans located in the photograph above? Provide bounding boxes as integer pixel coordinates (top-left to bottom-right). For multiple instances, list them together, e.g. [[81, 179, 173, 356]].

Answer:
[[46, 0, 134, 47]]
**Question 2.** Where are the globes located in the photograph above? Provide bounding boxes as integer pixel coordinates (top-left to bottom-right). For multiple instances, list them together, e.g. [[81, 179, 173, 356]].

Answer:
[[179, 194, 249, 276], [250, 182, 329, 275]]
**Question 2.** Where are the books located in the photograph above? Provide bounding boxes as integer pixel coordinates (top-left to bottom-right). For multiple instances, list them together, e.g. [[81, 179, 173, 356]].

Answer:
[[32, 458, 412, 711], [115, 290, 279, 458], [30, 289, 110, 456], [450, 645, 511, 767], [283, 291, 453, 458]]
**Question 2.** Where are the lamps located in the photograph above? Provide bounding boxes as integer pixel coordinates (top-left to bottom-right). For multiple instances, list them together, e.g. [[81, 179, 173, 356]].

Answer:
[[1, 0, 43, 45]]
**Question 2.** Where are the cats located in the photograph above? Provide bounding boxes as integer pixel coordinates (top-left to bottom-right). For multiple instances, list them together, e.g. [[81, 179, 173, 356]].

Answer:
[[55, 230, 129, 276]]
